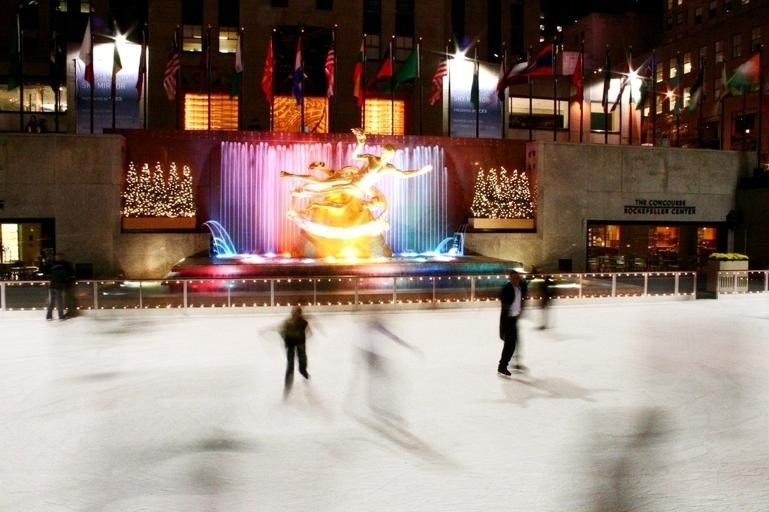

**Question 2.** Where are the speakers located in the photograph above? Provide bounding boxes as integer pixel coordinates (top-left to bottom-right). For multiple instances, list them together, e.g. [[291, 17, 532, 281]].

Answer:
[[75, 261, 94, 279], [559, 258, 572, 274]]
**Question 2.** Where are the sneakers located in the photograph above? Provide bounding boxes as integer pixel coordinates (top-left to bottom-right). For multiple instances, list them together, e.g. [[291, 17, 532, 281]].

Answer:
[[498, 368, 511, 376]]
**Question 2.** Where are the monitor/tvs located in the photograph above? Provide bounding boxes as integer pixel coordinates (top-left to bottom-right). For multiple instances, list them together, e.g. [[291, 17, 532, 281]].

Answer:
[[591, 112, 612, 132]]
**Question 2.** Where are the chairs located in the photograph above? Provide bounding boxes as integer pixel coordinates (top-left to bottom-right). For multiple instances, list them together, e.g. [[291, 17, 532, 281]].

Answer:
[[0, 260, 94, 281]]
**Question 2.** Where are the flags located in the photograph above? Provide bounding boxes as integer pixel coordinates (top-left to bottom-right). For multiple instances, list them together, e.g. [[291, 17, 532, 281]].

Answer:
[[199, 23, 336, 105], [668, 46, 763, 115], [0, 6, 185, 104], [511, 29, 656, 110], [350, 38, 511, 112]]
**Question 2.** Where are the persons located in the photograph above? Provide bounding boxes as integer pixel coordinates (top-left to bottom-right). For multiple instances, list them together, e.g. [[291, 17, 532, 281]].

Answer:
[[261, 303, 313, 400], [54, 252, 79, 318], [497, 268, 527, 377], [278, 127, 434, 215], [529, 267, 558, 331], [44, 259, 71, 320]]
